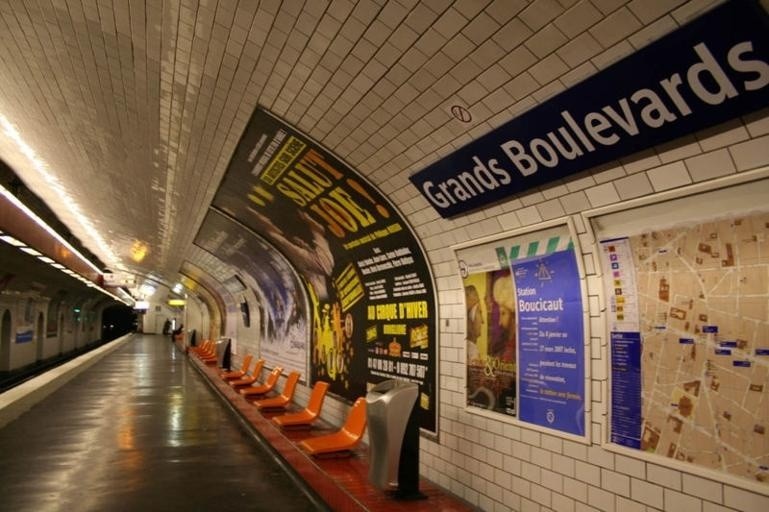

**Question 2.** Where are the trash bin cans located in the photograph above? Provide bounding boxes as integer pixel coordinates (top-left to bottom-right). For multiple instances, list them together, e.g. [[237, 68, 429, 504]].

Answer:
[[186, 328, 196, 347], [215, 338, 231, 368], [365, 381, 428, 501]]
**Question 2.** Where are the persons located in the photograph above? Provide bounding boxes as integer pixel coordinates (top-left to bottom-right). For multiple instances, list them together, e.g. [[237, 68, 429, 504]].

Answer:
[[244, 203, 337, 304], [464, 283, 505, 412], [489, 272, 517, 411], [171, 323, 185, 342], [162, 317, 171, 335]]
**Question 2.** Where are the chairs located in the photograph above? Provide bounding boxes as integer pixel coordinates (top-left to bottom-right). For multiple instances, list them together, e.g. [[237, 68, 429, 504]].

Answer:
[[189, 338, 368, 460]]
